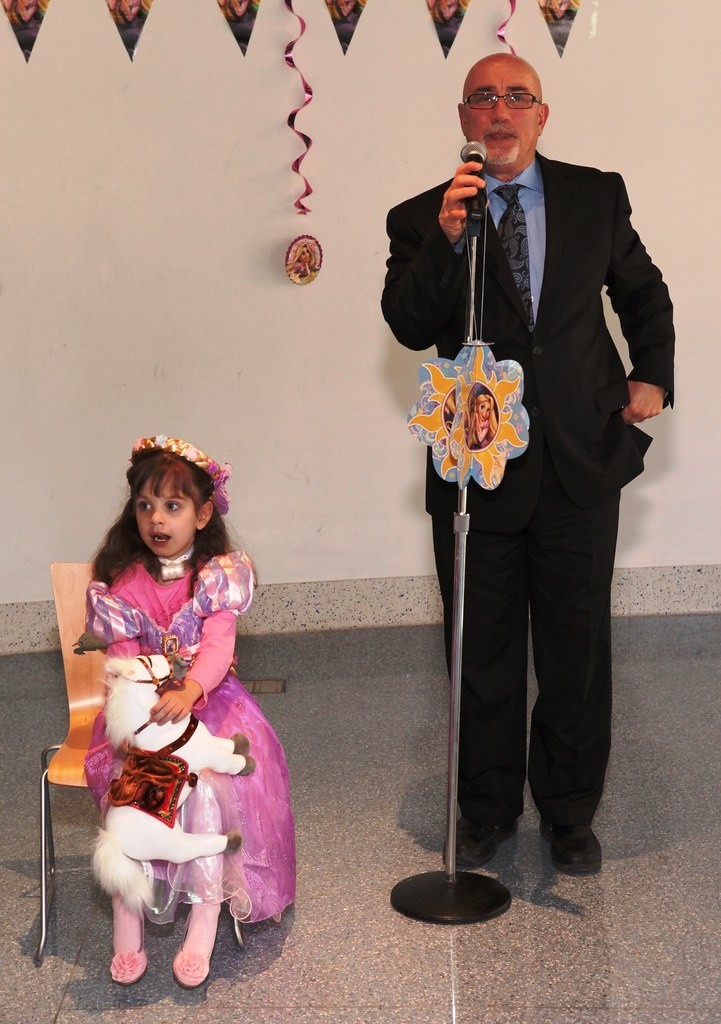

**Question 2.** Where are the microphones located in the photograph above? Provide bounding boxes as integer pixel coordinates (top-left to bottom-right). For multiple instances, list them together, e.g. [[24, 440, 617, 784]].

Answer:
[[460, 140, 487, 221]]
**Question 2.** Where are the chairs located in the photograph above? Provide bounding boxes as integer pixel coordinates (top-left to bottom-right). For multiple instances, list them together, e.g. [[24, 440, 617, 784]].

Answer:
[[36, 569, 245, 965]]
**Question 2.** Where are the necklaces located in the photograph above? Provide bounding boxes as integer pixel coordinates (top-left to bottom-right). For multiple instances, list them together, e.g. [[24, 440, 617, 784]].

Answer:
[[158, 545, 195, 581]]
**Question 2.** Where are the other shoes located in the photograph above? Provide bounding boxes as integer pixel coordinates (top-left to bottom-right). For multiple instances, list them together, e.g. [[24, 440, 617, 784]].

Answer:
[[109, 912, 150, 987], [173, 911, 212, 987]]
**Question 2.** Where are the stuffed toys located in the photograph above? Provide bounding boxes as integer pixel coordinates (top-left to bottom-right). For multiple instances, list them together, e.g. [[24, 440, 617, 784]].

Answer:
[[92, 653, 255, 917]]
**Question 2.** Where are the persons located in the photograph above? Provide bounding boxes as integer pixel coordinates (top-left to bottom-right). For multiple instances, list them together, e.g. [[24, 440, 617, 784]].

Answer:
[[79, 435, 297, 988], [380, 52, 675, 873]]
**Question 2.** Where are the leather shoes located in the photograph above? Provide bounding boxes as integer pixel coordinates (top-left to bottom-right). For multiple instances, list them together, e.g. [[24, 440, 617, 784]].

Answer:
[[540, 814, 602, 875], [442, 815, 519, 869]]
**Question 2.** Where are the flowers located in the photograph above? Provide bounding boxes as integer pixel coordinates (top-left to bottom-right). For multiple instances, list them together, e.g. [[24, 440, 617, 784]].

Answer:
[[210, 462, 234, 517]]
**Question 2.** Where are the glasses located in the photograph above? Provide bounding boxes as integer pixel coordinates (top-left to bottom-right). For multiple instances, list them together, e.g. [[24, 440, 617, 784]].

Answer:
[[463, 92, 542, 109]]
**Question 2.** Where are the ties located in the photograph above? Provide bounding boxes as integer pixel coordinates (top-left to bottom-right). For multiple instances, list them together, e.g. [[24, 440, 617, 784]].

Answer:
[[493, 185, 535, 334]]
